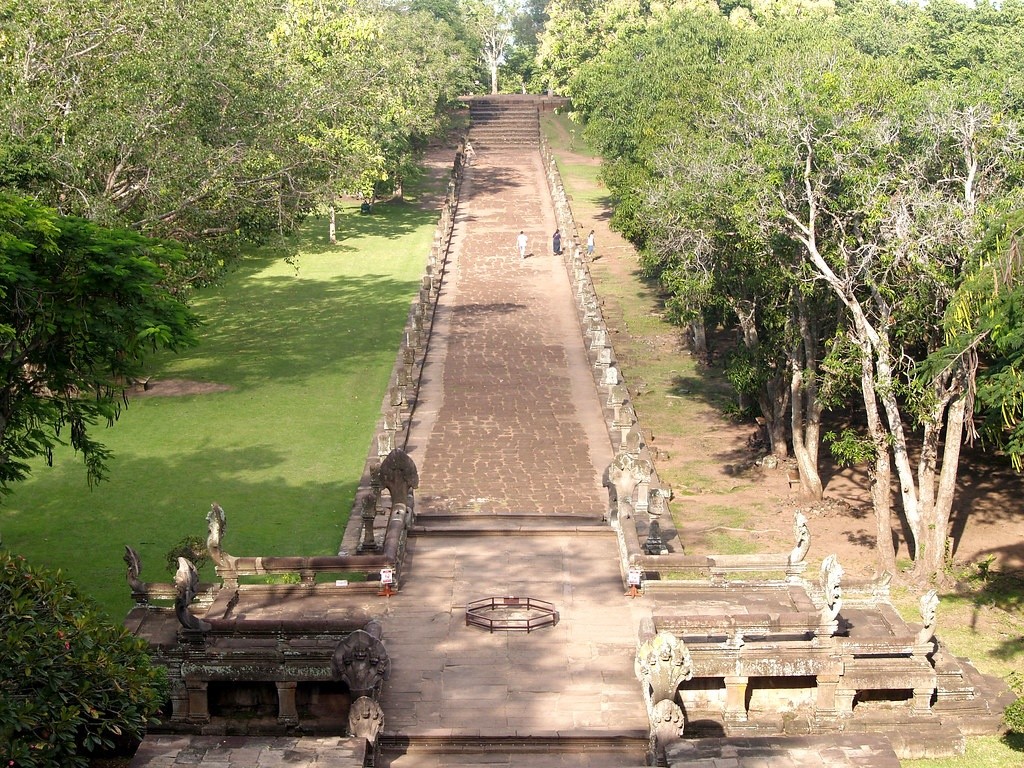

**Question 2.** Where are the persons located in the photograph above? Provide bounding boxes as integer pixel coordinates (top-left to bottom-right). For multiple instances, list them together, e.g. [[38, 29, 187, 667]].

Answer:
[[585, 230, 595, 257], [552, 229, 563, 256], [516, 231, 528, 259]]
